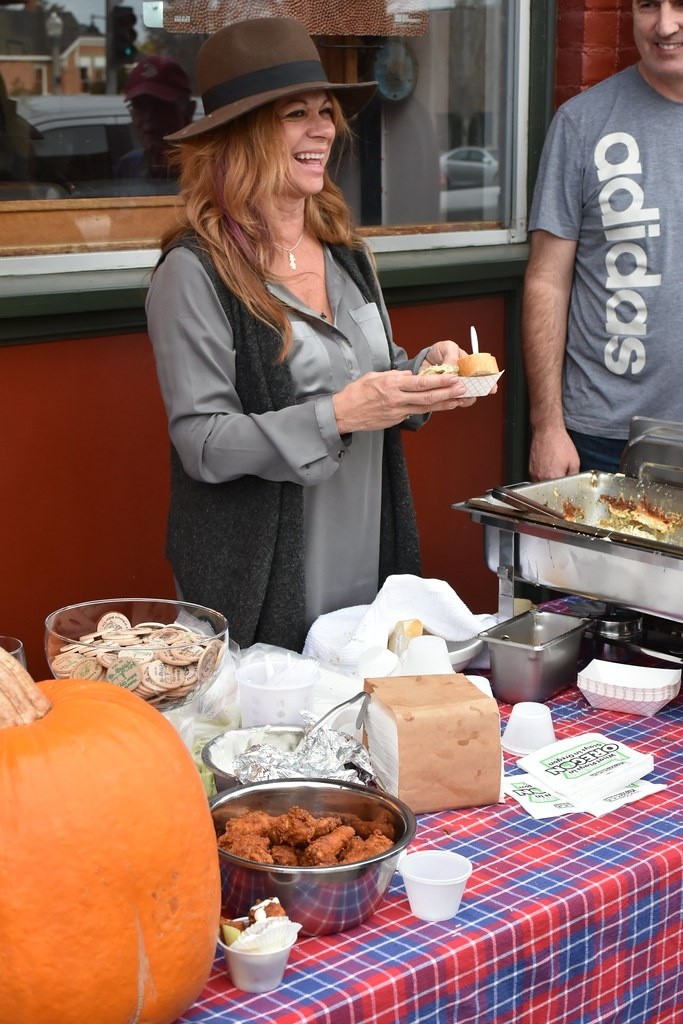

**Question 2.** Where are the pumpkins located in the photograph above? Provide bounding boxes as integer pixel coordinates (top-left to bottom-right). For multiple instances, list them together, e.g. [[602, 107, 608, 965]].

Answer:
[[0, 645, 222, 1024]]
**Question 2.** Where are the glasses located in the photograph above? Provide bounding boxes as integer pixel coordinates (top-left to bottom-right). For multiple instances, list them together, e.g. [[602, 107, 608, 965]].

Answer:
[[126, 101, 177, 114]]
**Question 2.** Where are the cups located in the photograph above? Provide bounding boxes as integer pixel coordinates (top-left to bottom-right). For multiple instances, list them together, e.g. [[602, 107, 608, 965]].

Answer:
[[234, 662, 316, 728], [218, 916, 298, 993], [0, 635, 27, 671], [397, 850, 473, 922], [501, 702, 556, 757]]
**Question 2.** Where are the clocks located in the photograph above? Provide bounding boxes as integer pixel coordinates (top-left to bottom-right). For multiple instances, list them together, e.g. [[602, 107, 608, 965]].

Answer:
[[372, 39, 420, 106]]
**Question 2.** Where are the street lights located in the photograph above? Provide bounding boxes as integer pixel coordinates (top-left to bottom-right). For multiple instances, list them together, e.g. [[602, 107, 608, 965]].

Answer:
[[44, 10, 64, 91]]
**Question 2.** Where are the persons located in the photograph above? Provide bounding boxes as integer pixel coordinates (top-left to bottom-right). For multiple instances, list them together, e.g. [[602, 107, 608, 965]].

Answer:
[[144, 18, 497, 653], [522, 0, 683, 483], [0, 72, 45, 201], [71, 55, 197, 199]]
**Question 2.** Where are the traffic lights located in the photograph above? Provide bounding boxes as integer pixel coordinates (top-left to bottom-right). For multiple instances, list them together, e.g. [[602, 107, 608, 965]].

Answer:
[[112, 6, 140, 65]]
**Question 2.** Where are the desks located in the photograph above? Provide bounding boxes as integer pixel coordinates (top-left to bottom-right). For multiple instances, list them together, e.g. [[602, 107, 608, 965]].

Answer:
[[177, 681, 683, 1024]]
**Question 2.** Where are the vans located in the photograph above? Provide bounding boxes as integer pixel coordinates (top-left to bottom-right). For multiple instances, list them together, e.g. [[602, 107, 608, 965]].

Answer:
[[8, 91, 207, 183]]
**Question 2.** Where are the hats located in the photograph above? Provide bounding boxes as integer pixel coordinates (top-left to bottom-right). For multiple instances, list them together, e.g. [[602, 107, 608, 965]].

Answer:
[[124, 55, 193, 102], [163, 18, 378, 142]]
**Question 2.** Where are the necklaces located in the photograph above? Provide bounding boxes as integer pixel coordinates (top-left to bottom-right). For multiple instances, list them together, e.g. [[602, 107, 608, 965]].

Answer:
[[272, 234, 303, 270]]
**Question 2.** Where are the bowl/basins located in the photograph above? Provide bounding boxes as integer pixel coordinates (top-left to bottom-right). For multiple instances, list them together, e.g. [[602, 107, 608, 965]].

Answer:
[[44, 598, 229, 713], [200, 725, 367, 794], [208, 778, 418, 938]]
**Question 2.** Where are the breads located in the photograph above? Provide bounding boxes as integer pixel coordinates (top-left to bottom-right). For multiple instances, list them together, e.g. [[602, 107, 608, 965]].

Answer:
[[417, 364, 458, 375], [457, 352, 499, 378], [388, 618, 424, 655]]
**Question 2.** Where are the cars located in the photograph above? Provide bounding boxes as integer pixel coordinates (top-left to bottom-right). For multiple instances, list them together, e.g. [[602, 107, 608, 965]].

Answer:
[[438, 146, 501, 188]]
